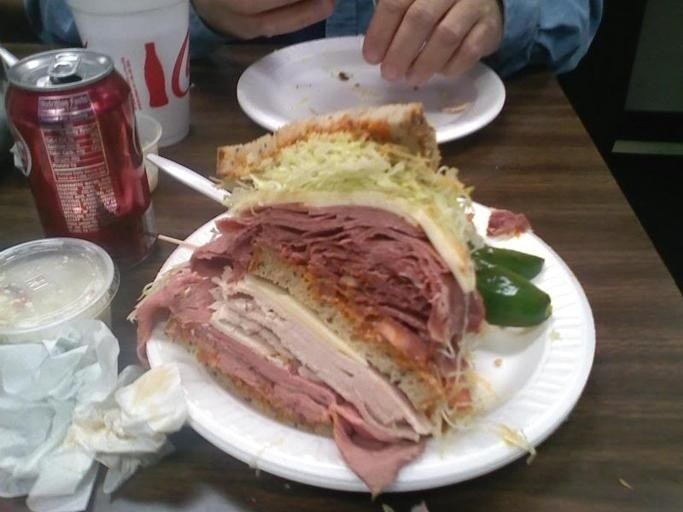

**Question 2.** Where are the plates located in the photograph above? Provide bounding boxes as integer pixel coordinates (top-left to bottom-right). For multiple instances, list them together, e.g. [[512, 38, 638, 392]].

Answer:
[[143, 196, 596, 497], [234, 34, 507, 146]]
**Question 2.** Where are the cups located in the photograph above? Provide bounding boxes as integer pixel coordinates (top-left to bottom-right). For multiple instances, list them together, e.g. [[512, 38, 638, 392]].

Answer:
[[63, 0, 189, 151], [0, 237, 120, 348], [138, 116, 161, 190]]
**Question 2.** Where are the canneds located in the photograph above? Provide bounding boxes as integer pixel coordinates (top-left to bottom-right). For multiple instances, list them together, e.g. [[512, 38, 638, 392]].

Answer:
[[6, 48, 158, 271]]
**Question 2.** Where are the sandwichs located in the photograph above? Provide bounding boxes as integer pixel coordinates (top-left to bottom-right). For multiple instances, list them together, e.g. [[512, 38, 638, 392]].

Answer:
[[128, 105, 485, 495]]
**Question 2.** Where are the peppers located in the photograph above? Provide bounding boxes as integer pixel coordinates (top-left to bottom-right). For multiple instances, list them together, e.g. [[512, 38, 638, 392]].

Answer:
[[470, 243, 552, 326]]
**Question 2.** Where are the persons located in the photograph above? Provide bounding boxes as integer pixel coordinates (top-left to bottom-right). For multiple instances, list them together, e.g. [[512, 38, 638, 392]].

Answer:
[[37, 0, 610, 86]]
